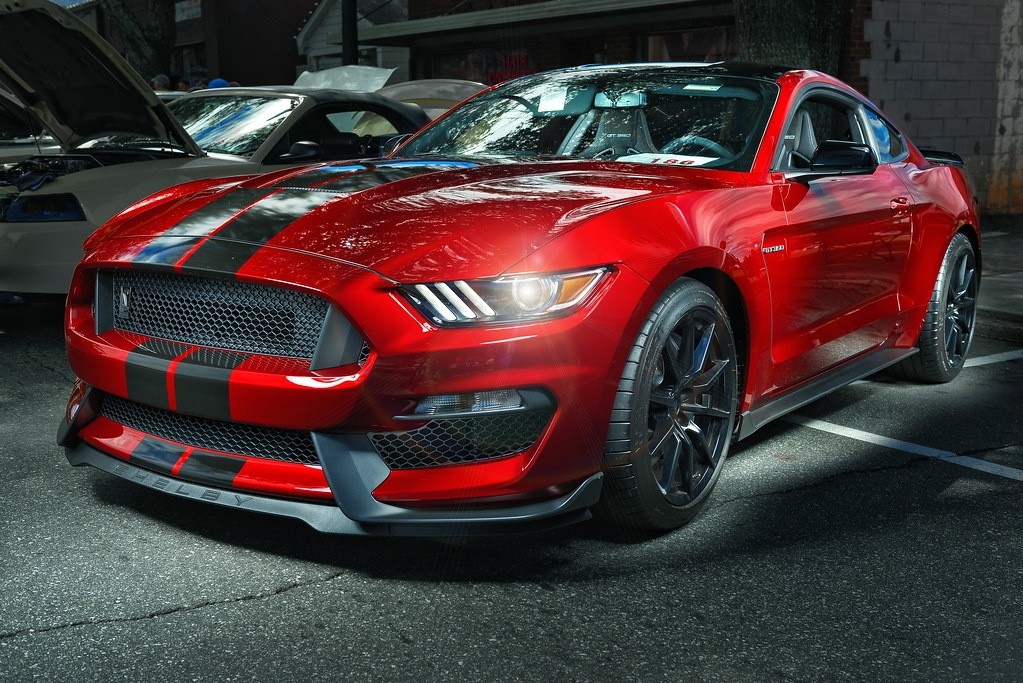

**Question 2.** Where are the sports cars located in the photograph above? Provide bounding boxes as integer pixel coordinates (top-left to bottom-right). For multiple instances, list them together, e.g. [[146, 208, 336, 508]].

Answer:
[[52, 58, 985, 544], [0, 0, 502, 317]]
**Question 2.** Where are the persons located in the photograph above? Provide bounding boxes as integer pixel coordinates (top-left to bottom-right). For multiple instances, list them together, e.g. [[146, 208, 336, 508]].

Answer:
[[151, 74, 171, 90], [178, 78, 190, 91], [229, 81, 241, 89], [208, 78, 229, 89], [188, 79, 210, 93]]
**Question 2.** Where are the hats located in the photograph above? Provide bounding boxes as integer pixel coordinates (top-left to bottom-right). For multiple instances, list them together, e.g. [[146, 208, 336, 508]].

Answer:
[[208, 78, 230, 89], [151, 74, 170, 86]]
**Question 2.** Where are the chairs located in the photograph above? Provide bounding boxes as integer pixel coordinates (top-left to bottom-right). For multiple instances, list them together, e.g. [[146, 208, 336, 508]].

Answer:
[[282, 131, 359, 157], [576, 108, 657, 158], [725, 99, 816, 163]]
